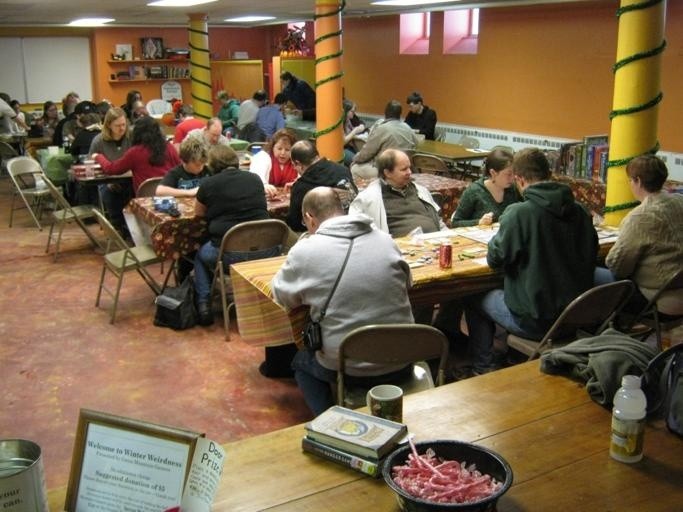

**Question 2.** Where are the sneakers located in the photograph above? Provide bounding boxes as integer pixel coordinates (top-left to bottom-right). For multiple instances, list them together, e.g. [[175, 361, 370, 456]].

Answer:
[[194, 303, 214, 327]]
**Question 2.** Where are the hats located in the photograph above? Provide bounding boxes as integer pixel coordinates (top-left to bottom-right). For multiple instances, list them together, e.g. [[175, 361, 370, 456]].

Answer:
[[74, 100, 98, 114]]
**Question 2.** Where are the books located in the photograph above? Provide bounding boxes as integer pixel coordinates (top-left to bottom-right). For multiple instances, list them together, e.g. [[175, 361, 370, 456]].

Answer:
[[113, 43, 133, 61], [108, 61, 192, 82], [546, 135, 609, 186], [165, 48, 190, 55], [301, 430, 416, 482], [303, 404, 409, 461], [165, 54, 191, 62], [140, 37, 163, 60]]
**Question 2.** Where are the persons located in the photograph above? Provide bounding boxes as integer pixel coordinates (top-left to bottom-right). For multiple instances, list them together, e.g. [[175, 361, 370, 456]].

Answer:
[[0, 88, 467, 346], [442, 149, 526, 229], [449, 146, 602, 382], [278, 70, 315, 121], [593, 153, 683, 328], [269, 184, 416, 418]]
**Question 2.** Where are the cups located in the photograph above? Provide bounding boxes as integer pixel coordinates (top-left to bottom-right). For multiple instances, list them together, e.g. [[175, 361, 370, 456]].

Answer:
[[63, 139, 69, 154], [251, 146, 261, 155], [365, 384, 402, 426], [83, 160, 94, 179]]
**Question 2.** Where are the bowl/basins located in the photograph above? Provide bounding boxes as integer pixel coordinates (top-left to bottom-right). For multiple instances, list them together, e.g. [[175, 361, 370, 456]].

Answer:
[[381, 439, 513, 512]]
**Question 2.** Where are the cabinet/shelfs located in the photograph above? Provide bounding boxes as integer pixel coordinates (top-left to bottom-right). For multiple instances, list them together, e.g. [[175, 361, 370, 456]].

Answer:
[[107, 59, 190, 82]]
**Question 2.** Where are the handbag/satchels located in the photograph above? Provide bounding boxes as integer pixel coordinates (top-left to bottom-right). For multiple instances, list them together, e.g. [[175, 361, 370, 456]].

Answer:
[[302, 322, 320, 351], [154, 287, 194, 329]]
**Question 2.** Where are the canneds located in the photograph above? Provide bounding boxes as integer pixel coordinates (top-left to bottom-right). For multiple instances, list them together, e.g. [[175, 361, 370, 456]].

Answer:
[[439, 244, 454, 268]]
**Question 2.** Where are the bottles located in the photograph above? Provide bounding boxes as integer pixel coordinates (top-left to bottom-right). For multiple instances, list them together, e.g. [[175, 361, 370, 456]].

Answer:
[[609, 375, 647, 464]]
